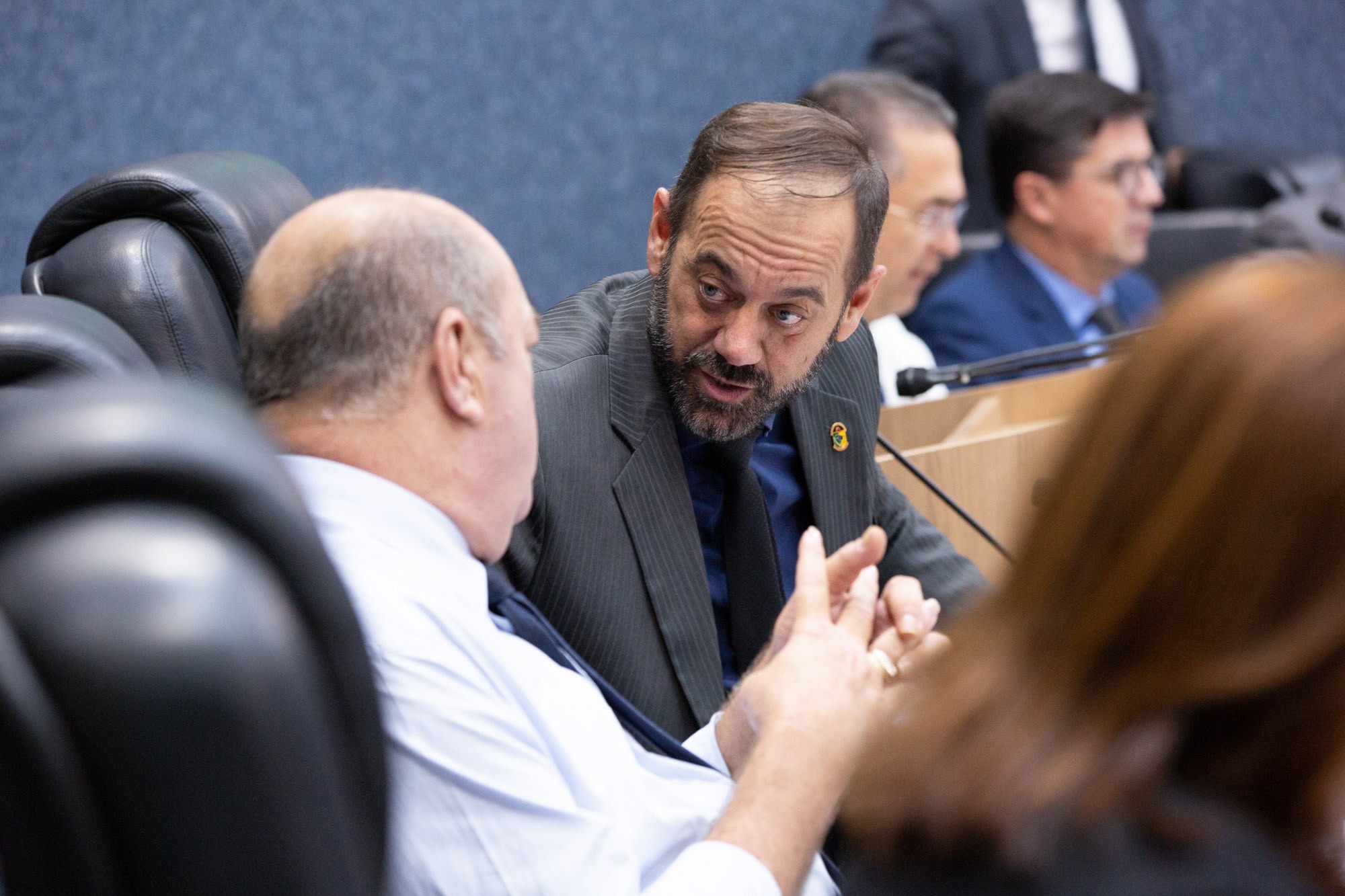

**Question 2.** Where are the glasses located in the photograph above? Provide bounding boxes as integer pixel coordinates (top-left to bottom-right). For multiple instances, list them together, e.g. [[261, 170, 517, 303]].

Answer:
[[1062, 155, 1164, 200], [887, 205, 969, 234]]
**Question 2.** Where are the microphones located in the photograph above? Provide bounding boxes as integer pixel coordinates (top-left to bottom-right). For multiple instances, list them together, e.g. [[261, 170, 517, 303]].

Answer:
[[892, 326, 1155, 400], [1320, 206, 1345, 237]]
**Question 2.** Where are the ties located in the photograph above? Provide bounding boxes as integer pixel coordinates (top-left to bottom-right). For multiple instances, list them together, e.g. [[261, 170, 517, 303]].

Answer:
[[703, 427, 784, 674], [1073, 0, 1097, 73], [487, 564, 848, 882]]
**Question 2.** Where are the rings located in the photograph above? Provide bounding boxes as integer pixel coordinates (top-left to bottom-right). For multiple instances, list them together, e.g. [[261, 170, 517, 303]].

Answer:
[[872, 647, 897, 682]]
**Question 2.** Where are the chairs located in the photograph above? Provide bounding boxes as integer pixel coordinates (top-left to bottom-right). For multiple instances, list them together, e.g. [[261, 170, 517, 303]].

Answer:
[[0, 148, 388, 896]]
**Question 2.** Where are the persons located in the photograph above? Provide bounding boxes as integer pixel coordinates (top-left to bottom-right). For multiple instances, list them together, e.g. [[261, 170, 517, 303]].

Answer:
[[899, 70, 1167, 389], [869, 0, 1163, 239], [798, 69, 972, 409], [818, 256, 1345, 896], [493, 99, 997, 744], [235, 186, 953, 896]]
